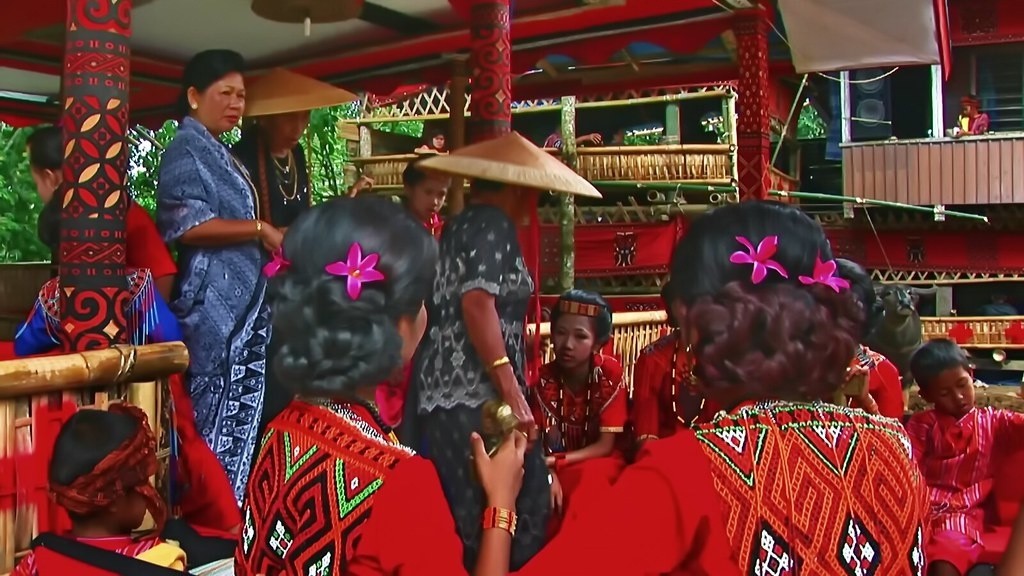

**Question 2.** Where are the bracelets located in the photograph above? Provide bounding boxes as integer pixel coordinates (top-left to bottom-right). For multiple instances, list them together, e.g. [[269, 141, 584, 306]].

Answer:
[[256, 220, 262, 234], [482, 507, 517, 538], [552, 452, 567, 471], [865, 399, 878, 413], [487, 356, 510, 371]]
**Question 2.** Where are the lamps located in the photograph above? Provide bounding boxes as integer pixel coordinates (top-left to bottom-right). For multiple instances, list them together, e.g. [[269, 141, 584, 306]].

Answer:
[[251, 0, 362, 35]]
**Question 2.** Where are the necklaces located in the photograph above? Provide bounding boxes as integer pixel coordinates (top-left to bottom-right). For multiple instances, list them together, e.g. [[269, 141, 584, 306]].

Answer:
[[430, 218, 434, 236], [671, 333, 705, 425], [557, 365, 591, 451], [270, 150, 307, 203], [355, 395, 400, 447]]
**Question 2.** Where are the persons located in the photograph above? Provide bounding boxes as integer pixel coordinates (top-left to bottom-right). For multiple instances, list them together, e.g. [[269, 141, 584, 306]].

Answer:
[[0, 50, 1024, 575], [543, 126, 601, 148], [956, 96, 989, 138], [420, 129, 449, 152]]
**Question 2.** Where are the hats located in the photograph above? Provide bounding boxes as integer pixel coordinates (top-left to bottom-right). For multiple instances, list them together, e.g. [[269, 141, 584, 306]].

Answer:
[[411, 130, 603, 200], [244, 66, 361, 117]]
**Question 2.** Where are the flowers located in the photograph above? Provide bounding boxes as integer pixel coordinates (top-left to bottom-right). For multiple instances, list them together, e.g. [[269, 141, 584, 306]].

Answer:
[[325, 242, 385, 299], [263, 246, 291, 278], [799, 255, 850, 293], [729, 235, 789, 285]]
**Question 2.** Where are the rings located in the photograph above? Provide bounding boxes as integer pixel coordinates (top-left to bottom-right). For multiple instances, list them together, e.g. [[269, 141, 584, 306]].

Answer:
[[517, 432, 527, 440]]
